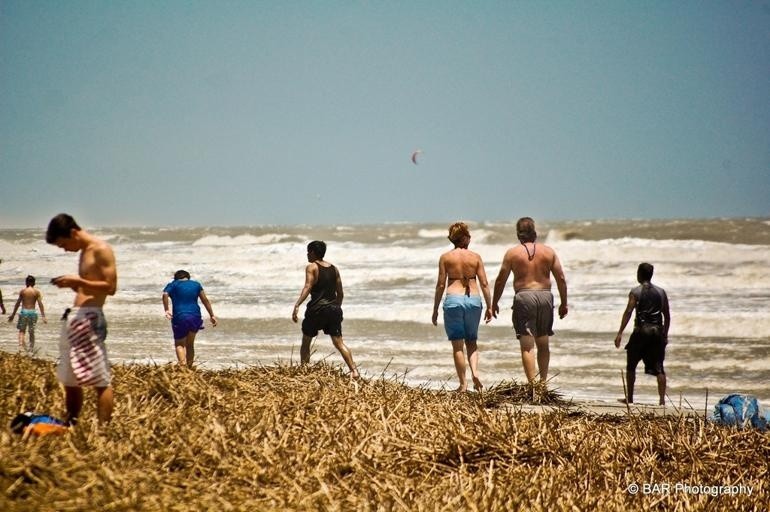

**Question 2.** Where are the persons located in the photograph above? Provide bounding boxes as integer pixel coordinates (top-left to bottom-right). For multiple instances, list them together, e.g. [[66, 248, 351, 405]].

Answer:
[[161, 270, 218, 370], [492, 215, 567, 394], [0, 260, 6, 314], [7, 275, 48, 354], [292, 241, 359, 378], [45, 213, 116, 436], [615, 263, 670, 406], [431, 222, 493, 395]]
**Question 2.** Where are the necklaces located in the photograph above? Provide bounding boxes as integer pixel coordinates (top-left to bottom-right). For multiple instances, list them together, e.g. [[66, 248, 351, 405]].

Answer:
[[522, 242, 536, 260]]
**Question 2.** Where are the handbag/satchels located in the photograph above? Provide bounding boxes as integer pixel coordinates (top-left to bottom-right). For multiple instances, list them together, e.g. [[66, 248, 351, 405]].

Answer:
[[707, 394, 767, 426], [11, 412, 69, 435]]
